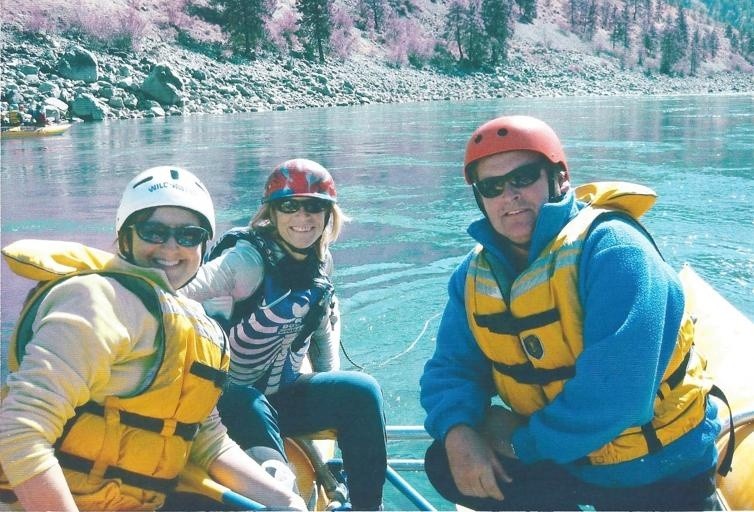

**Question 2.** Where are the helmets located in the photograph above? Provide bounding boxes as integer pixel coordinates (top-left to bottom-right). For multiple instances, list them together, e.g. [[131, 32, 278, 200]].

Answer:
[[463, 113, 572, 187], [113, 165, 216, 260], [261, 157, 338, 204]]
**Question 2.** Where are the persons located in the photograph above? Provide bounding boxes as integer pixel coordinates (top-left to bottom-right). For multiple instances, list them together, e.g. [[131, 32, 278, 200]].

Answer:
[[0, 166, 309, 512], [420, 115, 726, 512], [177, 155, 389, 511]]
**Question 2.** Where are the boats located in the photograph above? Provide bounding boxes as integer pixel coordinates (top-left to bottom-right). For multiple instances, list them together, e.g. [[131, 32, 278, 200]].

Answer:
[[175, 257, 753, 512], [0, 123, 74, 139]]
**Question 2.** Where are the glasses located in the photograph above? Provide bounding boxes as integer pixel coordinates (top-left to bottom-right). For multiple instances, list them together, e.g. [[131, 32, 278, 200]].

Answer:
[[125, 219, 209, 247], [271, 199, 329, 213], [475, 159, 548, 199]]
